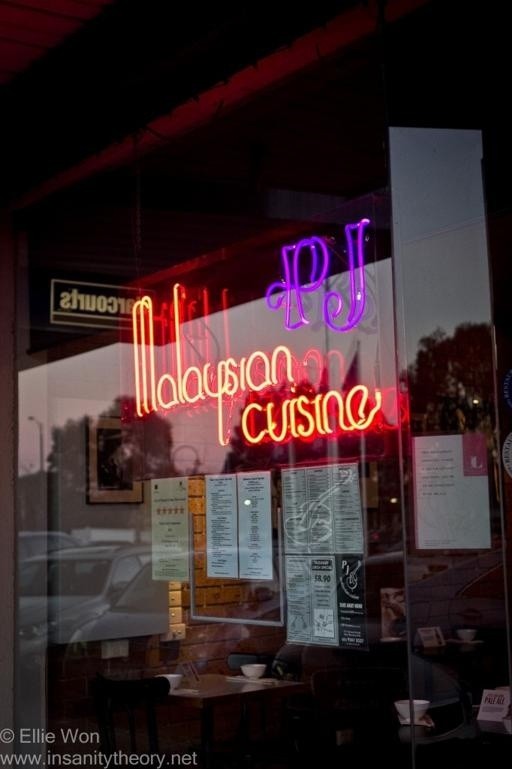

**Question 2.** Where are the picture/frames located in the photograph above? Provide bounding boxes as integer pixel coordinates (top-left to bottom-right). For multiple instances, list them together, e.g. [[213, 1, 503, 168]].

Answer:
[[85, 412, 145, 506]]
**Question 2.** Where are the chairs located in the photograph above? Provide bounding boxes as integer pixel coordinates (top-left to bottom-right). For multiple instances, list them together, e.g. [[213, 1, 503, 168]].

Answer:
[[94, 674, 171, 762]]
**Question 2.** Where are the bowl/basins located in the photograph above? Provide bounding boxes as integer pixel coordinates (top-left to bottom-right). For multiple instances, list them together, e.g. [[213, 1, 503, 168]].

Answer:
[[154, 673, 182, 692], [458, 630, 476, 640], [240, 663, 266, 679], [393, 699, 429, 723]]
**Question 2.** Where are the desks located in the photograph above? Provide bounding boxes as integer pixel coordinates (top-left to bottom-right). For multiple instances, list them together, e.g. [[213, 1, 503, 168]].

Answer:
[[153, 669, 309, 764]]
